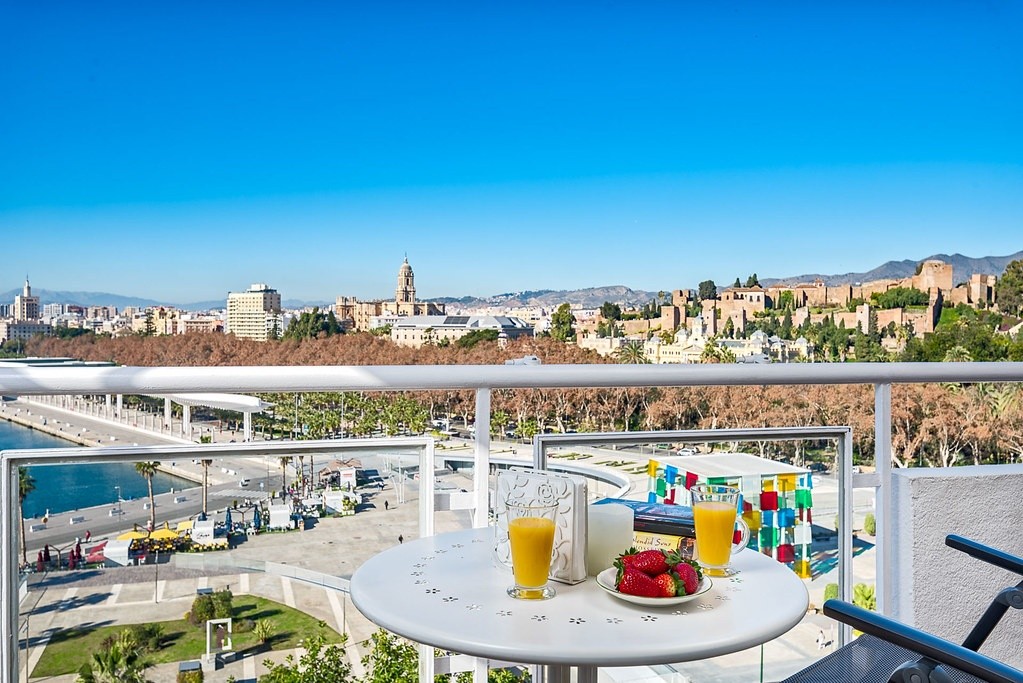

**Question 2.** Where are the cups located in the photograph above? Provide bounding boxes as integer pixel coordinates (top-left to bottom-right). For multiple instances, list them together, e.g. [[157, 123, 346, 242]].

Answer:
[[589, 504, 635, 576], [691, 485, 751, 575], [505, 497, 559, 601]]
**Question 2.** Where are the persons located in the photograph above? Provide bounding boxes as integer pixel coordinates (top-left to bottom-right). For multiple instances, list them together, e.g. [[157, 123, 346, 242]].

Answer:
[[85, 530, 90, 543], [385, 501, 388, 510], [818, 631, 825, 649], [399, 534, 403, 544], [378, 483, 384, 489]]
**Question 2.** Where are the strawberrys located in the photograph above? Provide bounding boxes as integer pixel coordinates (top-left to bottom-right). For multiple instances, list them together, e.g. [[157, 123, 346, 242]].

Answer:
[[612, 546, 703, 598]]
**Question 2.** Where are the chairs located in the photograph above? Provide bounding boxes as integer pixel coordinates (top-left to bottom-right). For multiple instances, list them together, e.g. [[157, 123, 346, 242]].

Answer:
[[779, 535, 1023, 683]]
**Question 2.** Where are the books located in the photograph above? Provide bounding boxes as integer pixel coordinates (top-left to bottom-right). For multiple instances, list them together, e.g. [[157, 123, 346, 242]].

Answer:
[[592, 498, 699, 560]]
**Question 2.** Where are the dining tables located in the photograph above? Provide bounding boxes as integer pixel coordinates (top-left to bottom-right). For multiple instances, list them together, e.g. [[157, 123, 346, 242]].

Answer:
[[349, 519, 809, 683]]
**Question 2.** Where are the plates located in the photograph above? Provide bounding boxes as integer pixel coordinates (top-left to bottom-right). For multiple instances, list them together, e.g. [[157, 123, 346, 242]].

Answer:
[[594, 567, 712, 608]]
